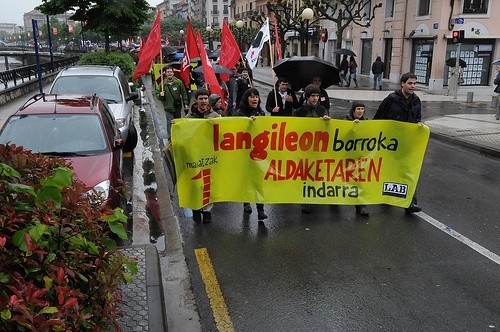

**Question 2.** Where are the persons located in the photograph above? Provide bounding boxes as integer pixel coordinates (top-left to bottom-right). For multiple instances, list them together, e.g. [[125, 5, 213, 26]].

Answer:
[[190, 57, 253, 116], [294, 84, 331, 211], [330, 102, 370, 216], [265, 77, 299, 115], [373, 72, 423, 214], [372, 56, 385, 90], [447, 65, 464, 96], [170, 88, 221, 223], [426, 61, 431, 86], [339, 54, 358, 88], [494, 65, 500, 120], [298, 77, 330, 116], [235, 88, 268, 220], [156, 66, 189, 145]]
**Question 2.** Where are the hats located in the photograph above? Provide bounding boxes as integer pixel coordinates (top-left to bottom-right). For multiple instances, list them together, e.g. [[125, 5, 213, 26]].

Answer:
[[209, 94, 221, 107]]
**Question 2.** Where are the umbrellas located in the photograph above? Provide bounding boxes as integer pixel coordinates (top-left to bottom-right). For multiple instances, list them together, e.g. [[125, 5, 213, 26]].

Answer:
[[273, 56, 339, 89], [162, 47, 234, 75], [492, 57, 500, 66], [445, 58, 467, 68], [332, 48, 356, 57]]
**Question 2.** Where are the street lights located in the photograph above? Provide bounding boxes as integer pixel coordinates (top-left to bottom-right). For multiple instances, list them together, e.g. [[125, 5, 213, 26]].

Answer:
[[302, 8, 314, 56], [180, 30, 184, 46], [206, 26, 211, 48], [18, 26, 24, 49], [236, 21, 243, 51]]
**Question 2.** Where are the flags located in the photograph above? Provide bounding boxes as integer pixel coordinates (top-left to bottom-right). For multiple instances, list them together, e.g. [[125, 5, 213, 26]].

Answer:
[[219, 19, 241, 82], [245, 17, 269, 70], [180, 17, 200, 88], [197, 30, 224, 106], [165, 35, 171, 46], [132, 8, 162, 80]]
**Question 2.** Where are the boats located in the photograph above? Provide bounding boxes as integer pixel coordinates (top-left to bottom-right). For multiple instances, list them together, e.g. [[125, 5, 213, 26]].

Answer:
[[0, 58, 20, 64]]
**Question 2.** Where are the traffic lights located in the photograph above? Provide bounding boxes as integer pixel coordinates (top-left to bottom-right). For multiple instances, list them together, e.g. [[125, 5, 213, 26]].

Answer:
[[460, 30, 465, 42], [453, 31, 459, 42]]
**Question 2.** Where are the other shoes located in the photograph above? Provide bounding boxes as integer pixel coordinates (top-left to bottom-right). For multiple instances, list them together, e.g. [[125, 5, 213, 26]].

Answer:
[[356, 208, 370, 215], [258, 211, 267, 220], [193, 210, 201, 223], [244, 203, 252, 212], [203, 212, 211, 223], [405, 204, 421, 213], [301, 204, 311, 213]]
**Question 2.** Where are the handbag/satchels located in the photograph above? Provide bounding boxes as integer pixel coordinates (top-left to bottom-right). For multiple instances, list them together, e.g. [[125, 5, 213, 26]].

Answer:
[[174, 98, 182, 109]]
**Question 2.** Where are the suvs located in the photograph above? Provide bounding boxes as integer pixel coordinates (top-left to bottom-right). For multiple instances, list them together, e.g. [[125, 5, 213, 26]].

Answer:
[[0, 92, 123, 235], [48, 63, 138, 132]]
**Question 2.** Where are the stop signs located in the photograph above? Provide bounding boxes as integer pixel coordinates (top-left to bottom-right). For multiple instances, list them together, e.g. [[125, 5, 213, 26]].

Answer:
[[321, 28, 326, 41]]
